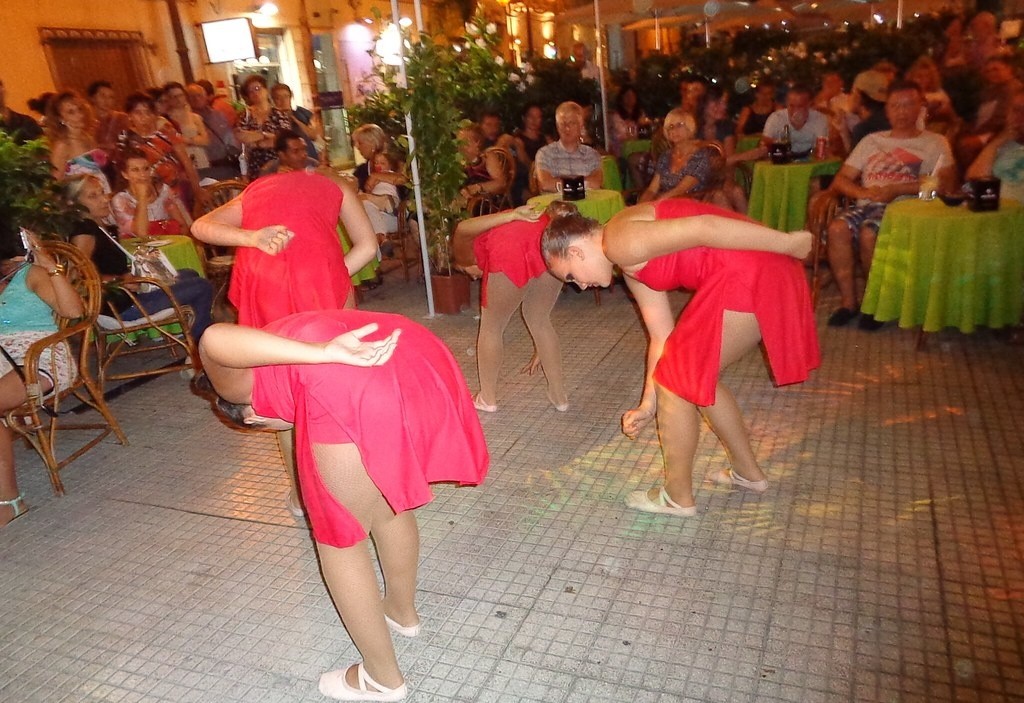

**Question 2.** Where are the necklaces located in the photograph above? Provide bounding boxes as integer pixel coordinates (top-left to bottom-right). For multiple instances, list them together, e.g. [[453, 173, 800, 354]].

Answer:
[[149, 189, 168, 230]]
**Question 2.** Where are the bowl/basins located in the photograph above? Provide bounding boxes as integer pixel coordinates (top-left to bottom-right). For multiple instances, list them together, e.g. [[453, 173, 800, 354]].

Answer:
[[937, 192, 968, 206]]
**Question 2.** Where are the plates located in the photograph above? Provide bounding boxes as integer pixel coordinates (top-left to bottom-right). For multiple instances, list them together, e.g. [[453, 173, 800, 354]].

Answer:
[[145, 239, 171, 247]]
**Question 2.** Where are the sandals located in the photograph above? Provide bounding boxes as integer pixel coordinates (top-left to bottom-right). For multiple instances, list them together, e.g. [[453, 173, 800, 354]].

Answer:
[[358, 276, 381, 291], [0, 495, 29, 527]]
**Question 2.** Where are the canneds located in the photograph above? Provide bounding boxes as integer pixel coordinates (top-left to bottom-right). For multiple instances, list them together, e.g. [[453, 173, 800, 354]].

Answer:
[[815, 136, 827, 160]]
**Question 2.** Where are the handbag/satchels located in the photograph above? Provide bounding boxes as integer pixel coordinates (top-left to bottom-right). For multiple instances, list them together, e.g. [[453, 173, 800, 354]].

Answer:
[[225, 143, 239, 163], [133, 250, 176, 293], [146, 219, 181, 236]]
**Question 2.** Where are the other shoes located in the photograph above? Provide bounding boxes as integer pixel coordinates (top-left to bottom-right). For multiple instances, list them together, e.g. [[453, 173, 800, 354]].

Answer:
[[858, 314, 881, 332], [625, 486, 696, 517], [708, 467, 768, 492], [318, 662, 409, 701], [385, 610, 420, 637], [286, 488, 307, 518], [545, 388, 568, 411], [471, 394, 498, 412], [828, 303, 861, 327]]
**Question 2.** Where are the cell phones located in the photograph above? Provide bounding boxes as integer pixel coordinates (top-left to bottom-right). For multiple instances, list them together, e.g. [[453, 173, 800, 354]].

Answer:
[[20, 231, 30, 249]]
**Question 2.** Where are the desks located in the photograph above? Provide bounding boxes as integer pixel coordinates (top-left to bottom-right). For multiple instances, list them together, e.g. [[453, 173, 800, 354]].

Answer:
[[528, 129, 1024, 350], [105, 237, 206, 340]]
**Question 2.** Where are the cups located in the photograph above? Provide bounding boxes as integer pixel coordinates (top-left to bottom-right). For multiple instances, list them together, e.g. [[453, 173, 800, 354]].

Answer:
[[811, 136, 832, 162], [919, 170, 936, 201], [556, 180, 587, 193]]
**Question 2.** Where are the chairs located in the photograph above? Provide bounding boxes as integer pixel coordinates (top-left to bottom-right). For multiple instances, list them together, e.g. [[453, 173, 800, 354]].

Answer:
[[193, 179, 252, 311], [22, 227, 215, 424], [378, 112, 851, 310], [1, 240, 127, 500]]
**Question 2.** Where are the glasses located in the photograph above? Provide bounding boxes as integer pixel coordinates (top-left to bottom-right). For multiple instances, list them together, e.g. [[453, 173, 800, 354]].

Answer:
[[666, 122, 684, 131], [248, 85, 262, 95]]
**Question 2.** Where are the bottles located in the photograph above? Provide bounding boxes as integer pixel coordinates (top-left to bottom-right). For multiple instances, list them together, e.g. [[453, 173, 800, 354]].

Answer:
[[237, 141, 249, 178], [782, 124, 791, 144]]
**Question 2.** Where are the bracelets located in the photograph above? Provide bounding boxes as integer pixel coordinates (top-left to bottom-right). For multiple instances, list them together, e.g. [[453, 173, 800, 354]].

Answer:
[[47, 268, 62, 277], [477, 183, 484, 191]]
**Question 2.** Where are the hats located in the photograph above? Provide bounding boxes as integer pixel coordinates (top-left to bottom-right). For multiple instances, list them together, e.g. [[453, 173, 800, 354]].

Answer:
[[855, 71, 890, 102]]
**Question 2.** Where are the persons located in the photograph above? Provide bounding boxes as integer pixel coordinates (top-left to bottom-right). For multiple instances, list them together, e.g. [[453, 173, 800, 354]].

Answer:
[[540, 198, 823, 519], [340, 123, 412, 293], [568, 0, 1024, 333], [1, 76, 320, 531], [188, 171, 489, 703], [458, 74, 746, 213], [448, 205, 568, 411]]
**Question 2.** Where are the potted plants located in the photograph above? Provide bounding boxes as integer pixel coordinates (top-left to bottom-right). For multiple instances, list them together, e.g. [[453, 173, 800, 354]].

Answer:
[[373, 6, 474, 316]]
